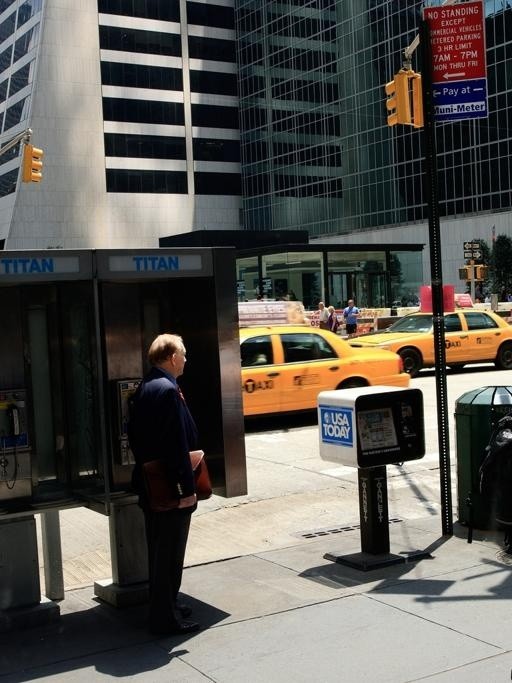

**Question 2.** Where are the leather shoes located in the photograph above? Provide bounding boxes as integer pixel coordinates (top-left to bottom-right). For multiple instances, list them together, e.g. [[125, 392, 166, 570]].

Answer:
[[156, 607, 200, 635]]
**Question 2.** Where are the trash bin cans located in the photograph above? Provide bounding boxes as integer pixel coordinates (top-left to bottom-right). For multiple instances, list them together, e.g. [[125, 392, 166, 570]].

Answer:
[[391, 306, 397, 316], [454, 386, 512, 531]]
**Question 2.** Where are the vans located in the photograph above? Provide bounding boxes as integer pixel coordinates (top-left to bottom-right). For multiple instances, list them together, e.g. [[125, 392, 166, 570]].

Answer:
[[311, 309, 344, 325], [230, 300, 411, 424]]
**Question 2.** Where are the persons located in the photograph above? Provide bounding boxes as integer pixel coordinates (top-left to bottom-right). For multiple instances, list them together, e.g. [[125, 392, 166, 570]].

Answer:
[[504, 292, 511, 301], [123, 331, 216, 639], [323, 304, 339, 333], [343, 299, 361, 333], [256, 293, 264, 301], [310, 300, 330, 330], [485, 292, 492, 302], [474, 295, 482, 302]]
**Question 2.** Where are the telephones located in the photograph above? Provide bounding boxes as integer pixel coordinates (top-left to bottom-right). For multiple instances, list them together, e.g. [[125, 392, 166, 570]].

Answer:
[[0, 389, 32, 454]]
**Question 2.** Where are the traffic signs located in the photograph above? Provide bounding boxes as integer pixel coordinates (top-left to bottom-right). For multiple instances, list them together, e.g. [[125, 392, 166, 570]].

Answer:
[[463, 241, 480, 249], [429, 78, 489, 121], [464, 251, 482, 258], [421, 2, 487, 82]]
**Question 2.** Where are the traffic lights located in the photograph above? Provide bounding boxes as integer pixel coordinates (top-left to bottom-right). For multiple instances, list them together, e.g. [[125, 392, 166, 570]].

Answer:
[[478, 265, 489, 280], [383, 69, 414, 126], [19, 144, 43, 184], [459, 268, 470, 280]]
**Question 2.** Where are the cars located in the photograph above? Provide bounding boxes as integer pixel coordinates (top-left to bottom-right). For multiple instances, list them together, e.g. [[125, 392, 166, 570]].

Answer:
[[346, 309, 512, 376]]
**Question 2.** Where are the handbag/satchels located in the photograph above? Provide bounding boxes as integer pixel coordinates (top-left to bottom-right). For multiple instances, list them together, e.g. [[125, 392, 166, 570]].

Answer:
[[144, 451, 212, 510]]
